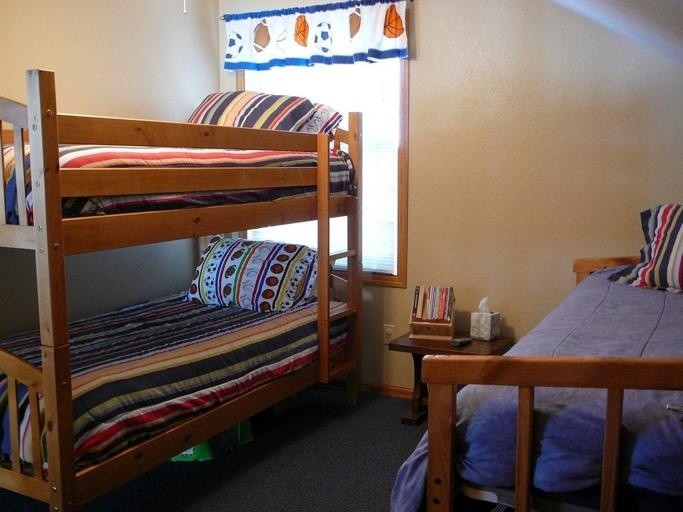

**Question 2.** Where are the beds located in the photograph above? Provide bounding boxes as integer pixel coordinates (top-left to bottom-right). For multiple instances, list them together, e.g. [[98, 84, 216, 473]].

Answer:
[[420, 255, 683, 511], [1, 69, 363, 511]]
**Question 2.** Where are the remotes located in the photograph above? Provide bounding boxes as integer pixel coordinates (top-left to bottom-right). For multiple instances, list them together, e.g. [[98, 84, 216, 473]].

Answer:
[[452, 337, 472, 347]]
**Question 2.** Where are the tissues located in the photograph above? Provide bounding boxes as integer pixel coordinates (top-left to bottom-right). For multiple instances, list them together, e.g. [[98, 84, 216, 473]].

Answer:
[[470, 296, 500, 342]]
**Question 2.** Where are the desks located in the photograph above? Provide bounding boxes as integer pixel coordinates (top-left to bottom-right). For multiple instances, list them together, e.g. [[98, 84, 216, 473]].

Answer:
[[388, 329, 515, 427]]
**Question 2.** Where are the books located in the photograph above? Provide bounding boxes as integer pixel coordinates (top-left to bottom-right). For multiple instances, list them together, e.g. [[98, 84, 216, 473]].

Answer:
[[412, 286, 455, 323]]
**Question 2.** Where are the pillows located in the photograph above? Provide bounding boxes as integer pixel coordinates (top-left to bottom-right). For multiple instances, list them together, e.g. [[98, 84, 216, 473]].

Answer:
[[607, 203, 683, 294], [183, 233, 335, 313], [186, 92, 344, 134]]
